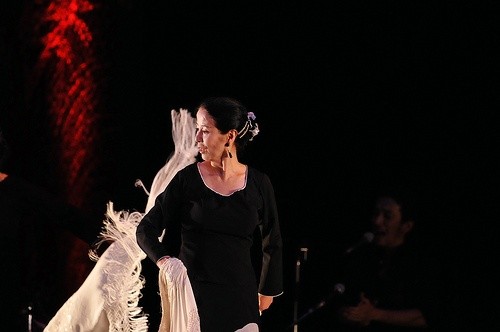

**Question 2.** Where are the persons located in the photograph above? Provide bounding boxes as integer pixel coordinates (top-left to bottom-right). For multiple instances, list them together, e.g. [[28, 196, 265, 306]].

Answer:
[[135, 98, 285, 332], [335, 186, 453, 332]]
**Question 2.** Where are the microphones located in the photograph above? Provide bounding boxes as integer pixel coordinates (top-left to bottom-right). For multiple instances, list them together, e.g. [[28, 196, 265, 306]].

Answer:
[[310, 283, 346, 312], [347, 232, 374, 252]]
